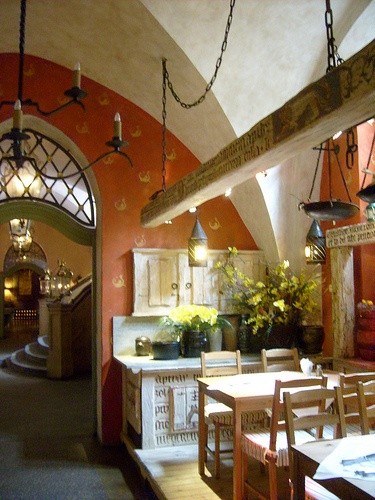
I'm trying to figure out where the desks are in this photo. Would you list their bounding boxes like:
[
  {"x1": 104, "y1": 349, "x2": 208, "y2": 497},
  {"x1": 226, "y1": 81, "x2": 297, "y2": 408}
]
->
[
  {"x1": 197, "y1": 370, "x2": 345, "y2": 500},
  {"x1": 290, "y1": 437, "x2": 375, "y2": 500},
  {"x1": 113, "y1": 354, "x2": 333, "y2": 450}
]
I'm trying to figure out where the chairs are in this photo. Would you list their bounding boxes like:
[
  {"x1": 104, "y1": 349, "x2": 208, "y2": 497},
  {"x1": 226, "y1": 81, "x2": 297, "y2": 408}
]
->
[{"x1": 194, "y1": 347, "x2": 375, "y2": 500}]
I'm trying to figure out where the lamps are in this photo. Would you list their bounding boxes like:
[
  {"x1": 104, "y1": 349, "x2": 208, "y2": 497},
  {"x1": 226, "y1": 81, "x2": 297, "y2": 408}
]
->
[
  {"x1": 186, "y1": 214, "x2": 210, "y2": 268},
  {"x1": 9, "y1": 218, "x2": 33, "y2": 259},
  {"x1": 0, "y1": 0, "x2": 135, "y2": 180},
  {"x1": 38, "y1": 257, "x2": 75, "y2": 297},
  {"x1": 304, "y1": 219, "x2": 328, "y2": 266}
]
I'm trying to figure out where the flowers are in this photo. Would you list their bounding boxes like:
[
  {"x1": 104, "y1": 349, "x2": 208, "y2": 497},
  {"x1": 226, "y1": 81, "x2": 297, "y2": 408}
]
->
[
  {"x1": 158, "y1": 303, "x2": 232, "y2": 331},
  {"x1": 211, "y1": 245, "x2": 332, "y2": 336}
]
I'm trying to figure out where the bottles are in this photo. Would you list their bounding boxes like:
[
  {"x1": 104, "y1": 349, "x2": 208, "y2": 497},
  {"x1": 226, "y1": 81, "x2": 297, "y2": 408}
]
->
[{"x1": 136, "y1": 336, "x2": 152, "y2": 356}]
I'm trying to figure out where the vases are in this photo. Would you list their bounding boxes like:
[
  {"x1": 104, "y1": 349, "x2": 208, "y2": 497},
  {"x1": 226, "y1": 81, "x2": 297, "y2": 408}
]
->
[
  {"x1": 265, "y1": 322, "x2": 293, "y2": 349},
  {"x1": 182, "y1": 328, "x2": 210, "y2": 359}
]
